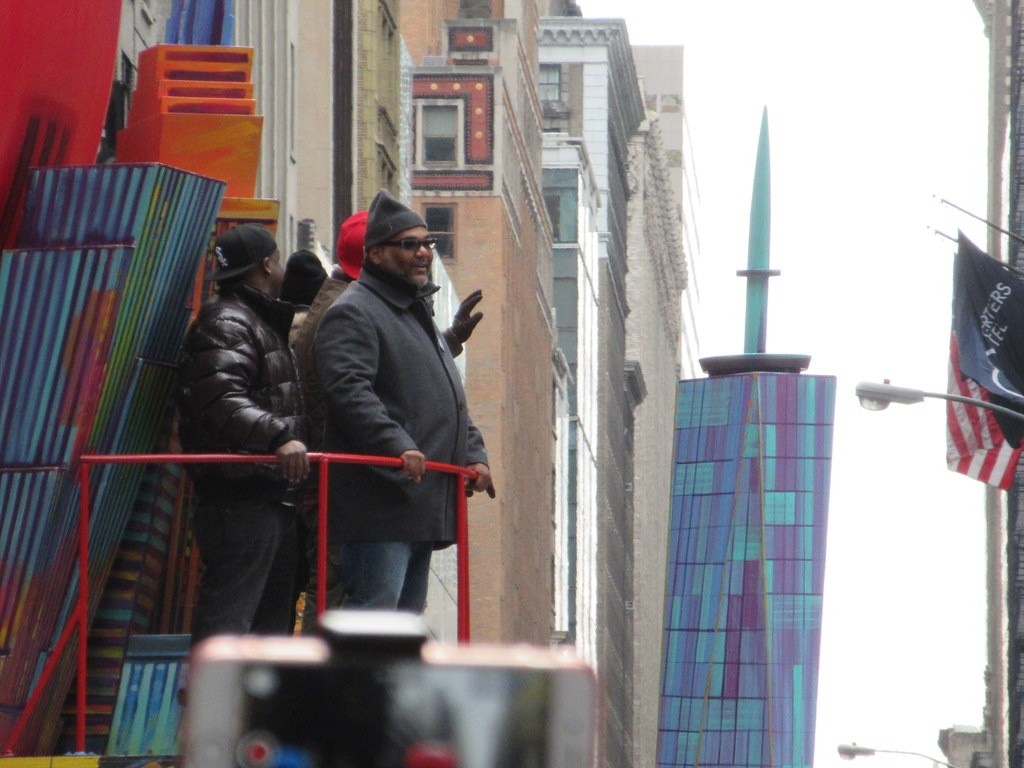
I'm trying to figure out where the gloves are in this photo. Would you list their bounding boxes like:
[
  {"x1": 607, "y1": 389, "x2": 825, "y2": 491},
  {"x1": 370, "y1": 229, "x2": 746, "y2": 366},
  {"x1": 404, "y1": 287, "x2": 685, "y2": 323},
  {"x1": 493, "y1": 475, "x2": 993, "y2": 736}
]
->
[{"x1": 451, "y1": 289, "x2": 483, "y2": 344}]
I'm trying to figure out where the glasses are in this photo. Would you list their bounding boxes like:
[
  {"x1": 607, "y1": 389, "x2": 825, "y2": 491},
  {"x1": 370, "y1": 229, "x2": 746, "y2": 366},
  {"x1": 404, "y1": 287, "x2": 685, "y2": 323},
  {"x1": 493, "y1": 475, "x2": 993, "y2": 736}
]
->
[{"x1": 387, "y1": 237, "x2": 439, "y2": 251}]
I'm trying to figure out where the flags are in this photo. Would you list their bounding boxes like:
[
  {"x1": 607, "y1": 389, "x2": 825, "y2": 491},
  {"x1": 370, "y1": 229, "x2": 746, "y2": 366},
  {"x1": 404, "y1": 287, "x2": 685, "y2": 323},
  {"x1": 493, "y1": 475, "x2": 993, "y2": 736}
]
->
[{"x1": 946, "y1": 231, "x2": 1024, "y2": 493}]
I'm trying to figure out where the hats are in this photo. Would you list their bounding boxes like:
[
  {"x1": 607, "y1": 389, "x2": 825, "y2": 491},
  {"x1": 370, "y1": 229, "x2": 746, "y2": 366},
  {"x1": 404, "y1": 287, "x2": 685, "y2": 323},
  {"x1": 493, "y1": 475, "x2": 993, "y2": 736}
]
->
[
  {"x1": 338, "y1": 210, "x2": 370, "y2": 279},
  {"x1": 204, "y1": 224, "x2": 277, "y2": 281},
  {"x1": 365, "y1": 188, "x2": 427, "y2": 251},
  {"x1": 279, "y1": 250, "x2": 329, "y2": 304}
]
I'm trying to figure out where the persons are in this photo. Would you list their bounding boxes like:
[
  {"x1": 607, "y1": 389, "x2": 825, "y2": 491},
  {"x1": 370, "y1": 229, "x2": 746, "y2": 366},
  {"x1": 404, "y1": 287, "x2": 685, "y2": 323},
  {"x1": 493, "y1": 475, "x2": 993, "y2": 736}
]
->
[{"x1": 174, "y1": 188, "x2": 492, "y2": 657}]
